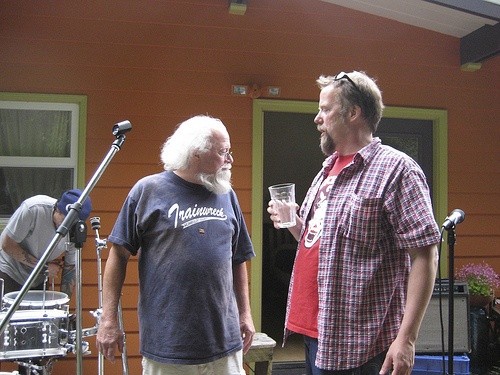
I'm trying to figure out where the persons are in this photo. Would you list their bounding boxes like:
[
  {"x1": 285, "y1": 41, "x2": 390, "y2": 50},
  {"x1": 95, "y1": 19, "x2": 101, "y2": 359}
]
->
[
  {"x1": 0, "y1": 189, "x2": 92, "y2": 295},
  {"x1": 95, "y1": 116, "x2": 257, "y2": 375},
  {"x1": 267, "y1": 71, "x2": 444, "y2": 375}
]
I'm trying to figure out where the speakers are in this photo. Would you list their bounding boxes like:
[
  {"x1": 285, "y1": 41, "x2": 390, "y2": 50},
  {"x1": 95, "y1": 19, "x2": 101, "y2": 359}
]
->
[{"x1": 414, "y1": 282, "x2": 472, "y2": 356}]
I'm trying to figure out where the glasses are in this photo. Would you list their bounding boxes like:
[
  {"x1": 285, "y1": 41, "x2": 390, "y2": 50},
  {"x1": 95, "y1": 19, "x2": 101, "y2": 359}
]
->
[
  {"x1": 216, "y1": 147, "x2": 233, "y2": 158},
  {"x1": 334, "y1": 71, "x2": 356, "y2": 88}
]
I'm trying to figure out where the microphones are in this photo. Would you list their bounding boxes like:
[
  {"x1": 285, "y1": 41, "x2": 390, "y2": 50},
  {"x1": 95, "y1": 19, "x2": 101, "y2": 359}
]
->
[{"x1": 443, "y1": 209, "x2": 466, "y2": 229}]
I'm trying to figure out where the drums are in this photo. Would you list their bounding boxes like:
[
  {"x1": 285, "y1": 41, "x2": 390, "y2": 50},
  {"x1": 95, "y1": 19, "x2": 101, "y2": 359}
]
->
[
  {"x1": 0, "y1": 308, "x2": 77, "y2": 358},
  {"x1": 2, "y1": 288, "x2": 70, "y2": 310}
]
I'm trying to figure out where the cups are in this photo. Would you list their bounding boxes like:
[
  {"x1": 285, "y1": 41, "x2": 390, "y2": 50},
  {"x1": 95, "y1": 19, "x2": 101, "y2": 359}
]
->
[{"x1": 268, "y1": 183, "x2": 297, "y2": 228}]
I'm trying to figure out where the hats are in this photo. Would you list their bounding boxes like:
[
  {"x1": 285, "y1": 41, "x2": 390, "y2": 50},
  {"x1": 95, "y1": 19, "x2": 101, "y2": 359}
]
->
[{"x1": 56, "y1": 189, "x2": 92, "y2": 220}]
